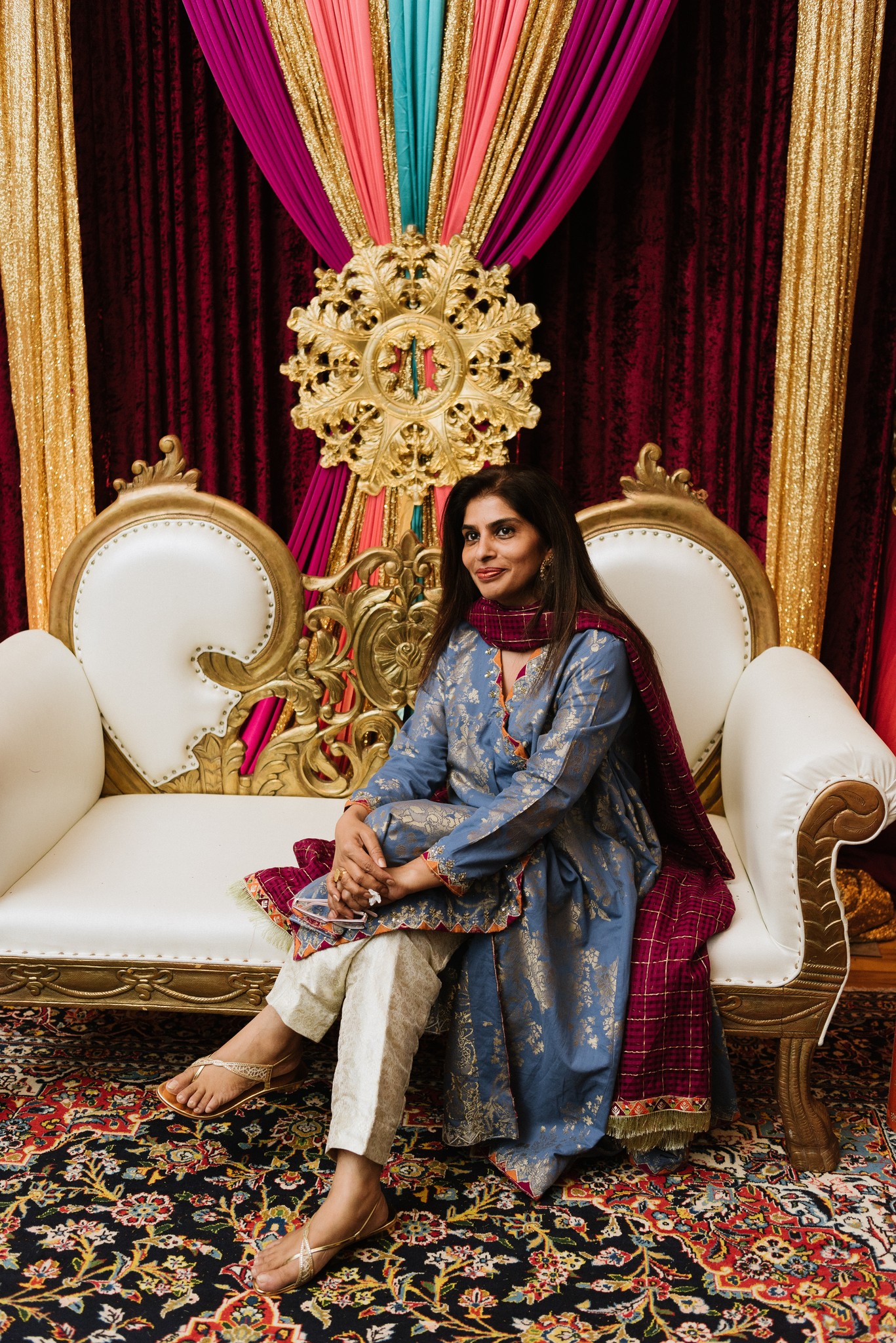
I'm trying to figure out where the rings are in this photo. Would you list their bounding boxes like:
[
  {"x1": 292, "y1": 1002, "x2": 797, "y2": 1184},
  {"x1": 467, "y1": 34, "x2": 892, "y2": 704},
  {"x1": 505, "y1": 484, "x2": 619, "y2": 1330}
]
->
[{"x1": 334, "y1": 867, "x2": 349, "y2": 882}]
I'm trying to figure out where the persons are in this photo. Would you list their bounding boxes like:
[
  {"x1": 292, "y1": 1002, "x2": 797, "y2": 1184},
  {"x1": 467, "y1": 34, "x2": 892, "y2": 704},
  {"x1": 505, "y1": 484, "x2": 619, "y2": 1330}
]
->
[{"x1": 158, "y1": 467, "x2": 737, "y2": 1293}]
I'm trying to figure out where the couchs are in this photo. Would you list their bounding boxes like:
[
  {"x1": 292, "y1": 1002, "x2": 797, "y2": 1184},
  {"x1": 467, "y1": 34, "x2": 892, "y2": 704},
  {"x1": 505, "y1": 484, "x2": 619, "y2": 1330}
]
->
[{"x1": 0, "y1": 431, "x2": 896, "y2": 1178}]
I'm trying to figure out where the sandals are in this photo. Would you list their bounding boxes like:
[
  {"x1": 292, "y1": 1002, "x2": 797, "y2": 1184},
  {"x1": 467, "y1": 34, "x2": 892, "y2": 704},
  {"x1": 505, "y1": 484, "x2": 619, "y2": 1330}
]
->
[
  {"x1": 255, "y1": 1192, "x2": 397, "y2": 1297},
  {"x1": 157, "y1": 1046, "x2": 303, "y2": 1119}
]
